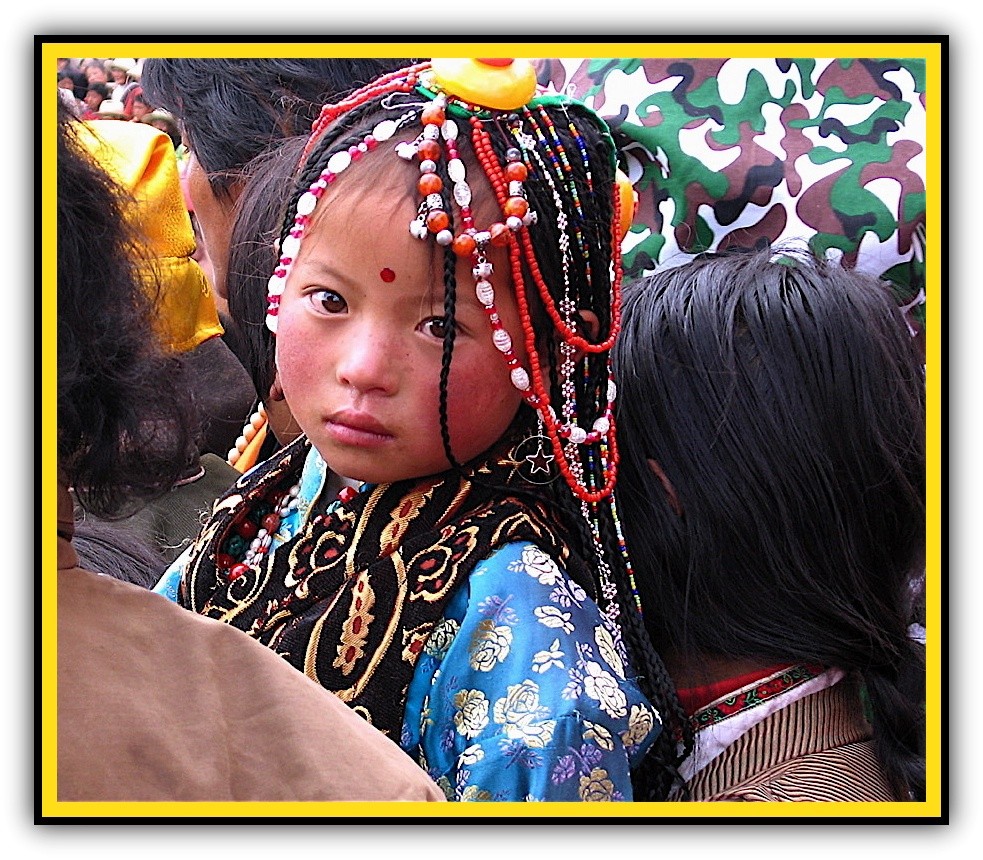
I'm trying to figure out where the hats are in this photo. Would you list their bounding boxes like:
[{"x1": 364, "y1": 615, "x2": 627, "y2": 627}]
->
[
  {"x1": 125, "y1": 58, "x2": 148, "y2": 80},
  {"x1": 103, "y1": 58, "x2": 137, "y2": 72},
  {"x1": 141, "y1": 108, "x2": 177, "y2": 133},
  {"x1": 94, "y1": 100, "x2": 131, "y2": 120}
]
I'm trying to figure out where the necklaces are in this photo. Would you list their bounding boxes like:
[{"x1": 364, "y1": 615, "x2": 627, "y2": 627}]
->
[
  {"x1": 225, "y1": 403, "x2": 270, "y2": 467},
  {"x1": 214, "y1": 469, "x2": 380, "y2": 579}
]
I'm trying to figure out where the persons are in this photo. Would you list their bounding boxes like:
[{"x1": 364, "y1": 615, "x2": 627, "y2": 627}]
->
[
  {"x1": 56, "y1": 56, "x2": 692, "y2": 803},
  {"x1": 606, "y1": 246, "x2": 926, "y2": 802}
]
